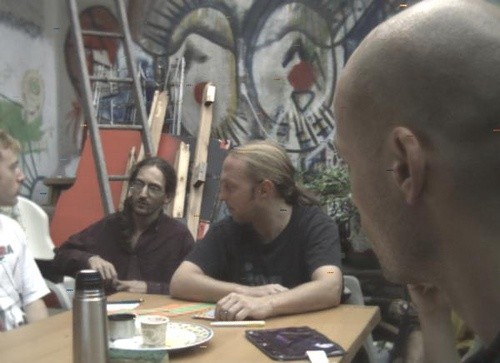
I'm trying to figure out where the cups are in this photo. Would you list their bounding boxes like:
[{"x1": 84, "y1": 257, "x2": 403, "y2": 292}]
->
[
  {"x1": 140, "y1": 314, "x2": 168, "y2": 348},
  {"x1": 107, "y1": 314, "x2": 136, "y2": 340}
]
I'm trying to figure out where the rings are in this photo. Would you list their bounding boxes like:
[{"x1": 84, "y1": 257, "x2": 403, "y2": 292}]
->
[{"x1": 220, "y1": 311, "x2": 227, "y2": 314}]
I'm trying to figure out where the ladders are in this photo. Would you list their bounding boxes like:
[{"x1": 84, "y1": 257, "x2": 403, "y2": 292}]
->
[{"x1": 68, "y1": 0, "x2": 157, "y2": 216}]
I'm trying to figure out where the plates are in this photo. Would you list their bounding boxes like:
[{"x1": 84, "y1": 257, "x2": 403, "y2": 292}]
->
[{"x1": 108, "y1": 322, "x2": 214, "y2": 352}]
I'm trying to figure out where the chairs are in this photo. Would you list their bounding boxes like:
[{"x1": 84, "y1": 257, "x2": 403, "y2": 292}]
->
[
  {"x1": 340, "y1": 273, "x2": 390, "y2": 363},
  {"x1": 9, "y1": 192, "x2": 77, "y2": 310}
]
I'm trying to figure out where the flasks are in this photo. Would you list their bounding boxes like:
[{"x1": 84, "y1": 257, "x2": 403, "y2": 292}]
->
[{"x1": 71, "y1": 269, "x2": 108, "y2": 363}]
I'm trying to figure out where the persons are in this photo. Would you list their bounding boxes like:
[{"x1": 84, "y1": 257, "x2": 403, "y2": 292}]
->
[
  {"x1": 56, "y1": 155, "x2": 195, "y2": 296},
  {"x1": 331, "y1": 0, "x2": 500, "y2": 363},
  {"x1": 0, "y1": 134, "x2": 52, "y2": 332},
  {"x1": 169, "y1": 140, "x2": 343, "y2": 321}
]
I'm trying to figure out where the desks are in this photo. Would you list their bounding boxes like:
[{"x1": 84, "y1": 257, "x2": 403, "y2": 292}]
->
[{"x1": 0, "y1": 283, "x2": 381, "y2": 363}]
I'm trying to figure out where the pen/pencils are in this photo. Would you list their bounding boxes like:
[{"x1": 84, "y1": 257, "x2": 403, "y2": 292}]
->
[
  {"x1": 210, "y1": 320, "x2": 265, "y2": 328},
  {"x1": 107, "y1": 298, "x2": 144, "y2": 304}
]
